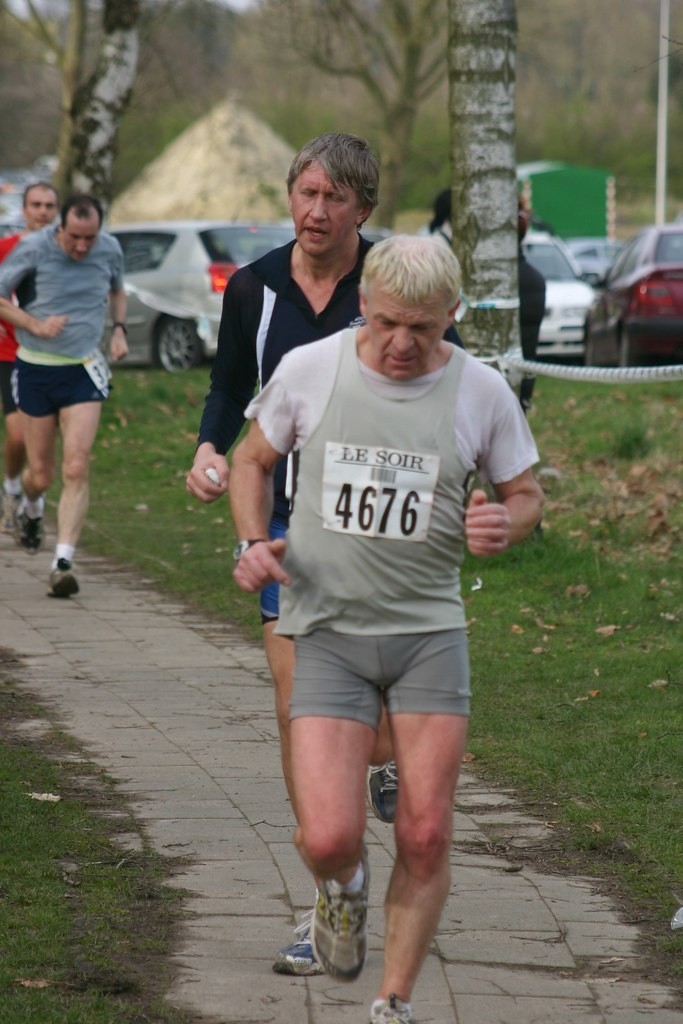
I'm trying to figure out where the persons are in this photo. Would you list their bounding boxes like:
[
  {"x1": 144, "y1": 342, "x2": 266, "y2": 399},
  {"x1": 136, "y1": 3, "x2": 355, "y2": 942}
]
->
[
  {"x1": 0, "y1": 181, "x2": 128, "y2": 598},
  {"x1": 419, "y1": 187, "x2": 547, "y2": 416},
  {"x1": 183, "y1": 136, "x2": 398, "y2": 977},
  {"x1": 224, "y1": 232, "x2": 548, "y2": 1024}
]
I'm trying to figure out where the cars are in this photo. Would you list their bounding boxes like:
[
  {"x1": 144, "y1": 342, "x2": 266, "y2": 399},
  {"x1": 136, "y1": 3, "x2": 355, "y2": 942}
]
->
[
  {"x1": 98, "y1": 222, "x2": 298, "y2": 373},
  {"x1": 521, "y1": 228, "x2": 628, "y2": 356},
  {"x1": 576, "y1": 225, "x2": 683, "y2": 368}
]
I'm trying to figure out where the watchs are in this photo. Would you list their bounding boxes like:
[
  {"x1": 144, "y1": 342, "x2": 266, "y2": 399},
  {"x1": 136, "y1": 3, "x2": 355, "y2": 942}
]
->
[{"x1": 233, "y1": 539, "x2": 266, "y2": 561}]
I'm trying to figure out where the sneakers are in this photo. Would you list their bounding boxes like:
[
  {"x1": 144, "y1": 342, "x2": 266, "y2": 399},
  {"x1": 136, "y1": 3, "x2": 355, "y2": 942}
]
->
[
  {"x1": 312, "y1": 845, "x2": 367, "y2": 983},
  {"x1": 272, "y1": 887, "x2": 326, "y2": 975},
  {"x1": 366, "y1": 758, "x2": 399, "y2": 822},
  {"x1": 20, "y1": 495, "x2": 45, "y2": 555},
  {"x1": 47, "y1": 558, "x2": 80, "y2": 598},
  {"x1": 0, "y1": 480, "x2": 22, "y2": 533},
  {"x1": 370, "y1": 993, "x2": 411, "y2": 1024}
]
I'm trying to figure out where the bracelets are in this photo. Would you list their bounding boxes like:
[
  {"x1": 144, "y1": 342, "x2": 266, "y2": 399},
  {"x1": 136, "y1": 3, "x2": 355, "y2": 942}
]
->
[{"x1": 114, "y1": 322, "x2": 127, "y2": 335}]
